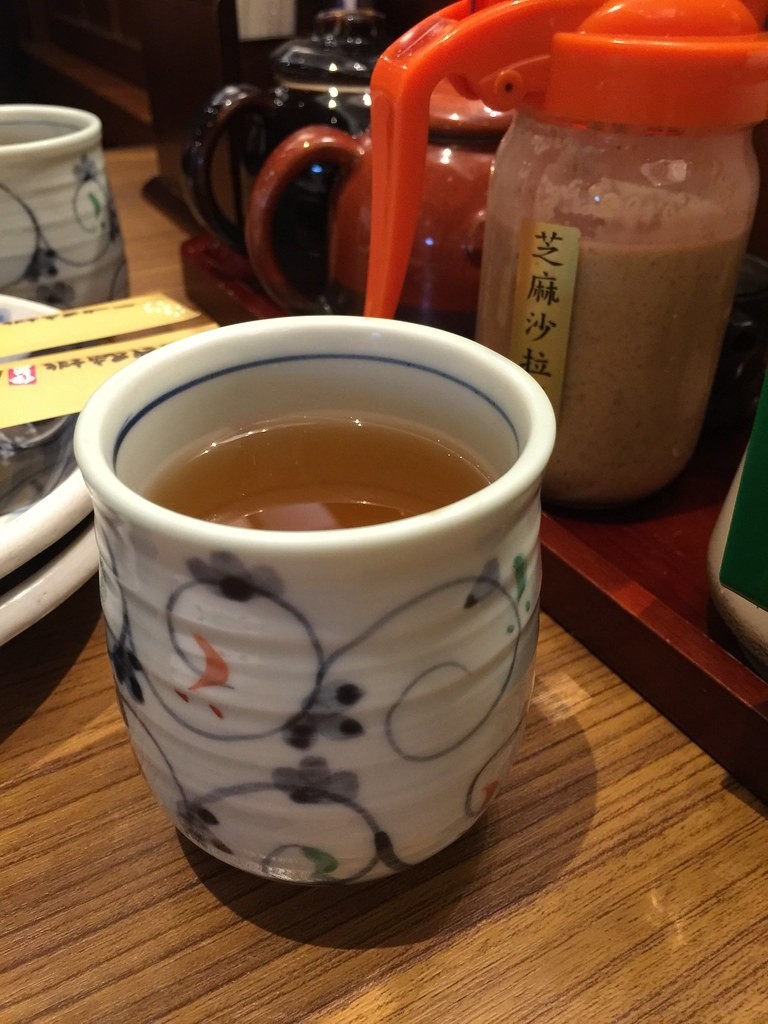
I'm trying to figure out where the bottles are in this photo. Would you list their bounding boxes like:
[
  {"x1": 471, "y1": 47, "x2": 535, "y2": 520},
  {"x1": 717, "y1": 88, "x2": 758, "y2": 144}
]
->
[{"x1": 357, "y1": 3, "x2": 767, "y2": 512}]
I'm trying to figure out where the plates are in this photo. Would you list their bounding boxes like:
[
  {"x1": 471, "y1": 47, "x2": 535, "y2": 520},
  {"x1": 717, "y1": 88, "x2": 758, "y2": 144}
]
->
[{"x1": 2, "y1": 289, "x2": 131, "y2": 645}]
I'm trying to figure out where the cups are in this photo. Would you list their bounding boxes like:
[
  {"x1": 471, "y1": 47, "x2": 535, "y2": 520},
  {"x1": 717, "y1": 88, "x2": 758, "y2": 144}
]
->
[
  {"x1": 4, "y1": 101, "x2": 126, "y2": 313},
  {"x1": 74, "y1": 304, "x2": 570, "y2": 890}
]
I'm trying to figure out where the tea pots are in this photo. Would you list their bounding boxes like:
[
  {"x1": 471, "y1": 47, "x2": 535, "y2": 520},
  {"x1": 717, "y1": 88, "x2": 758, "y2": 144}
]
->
[{"x1": 170, "y1": 7, "x2": 515, "y2": 330}]
